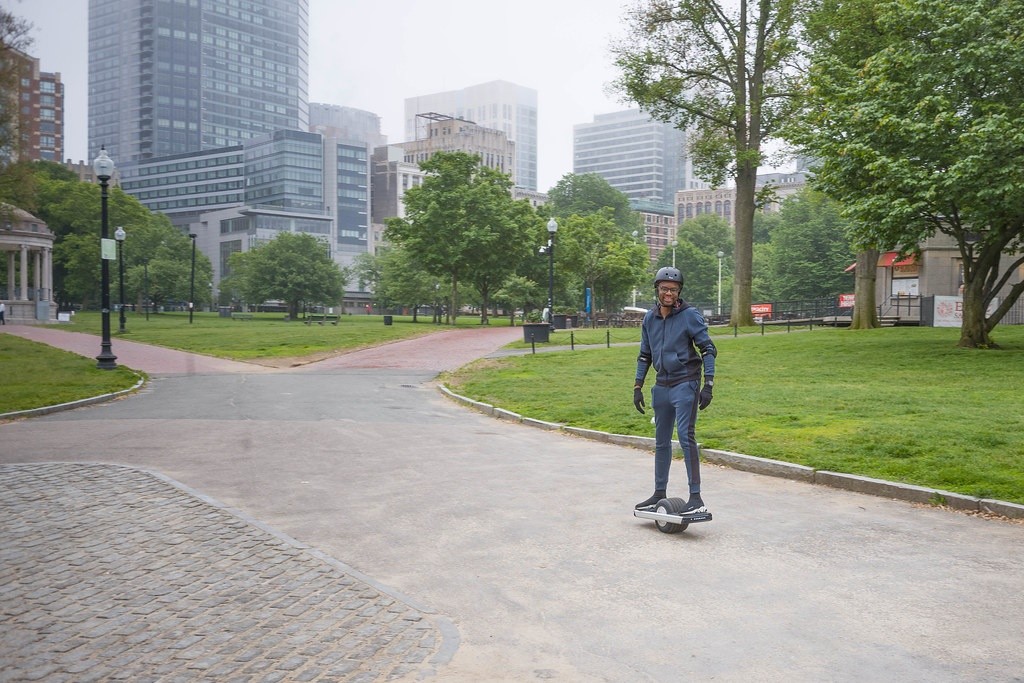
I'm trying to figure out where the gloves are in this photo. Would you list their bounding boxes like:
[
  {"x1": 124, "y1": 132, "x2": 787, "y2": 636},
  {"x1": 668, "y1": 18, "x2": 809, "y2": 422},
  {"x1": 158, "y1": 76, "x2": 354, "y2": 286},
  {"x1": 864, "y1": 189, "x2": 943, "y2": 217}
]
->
[
  {"x1": 699, "y1": 385, "x2": 714, "y2": 410},
  {"x1": 633, "y1": 388, "x2": 646, "y2": 414}
]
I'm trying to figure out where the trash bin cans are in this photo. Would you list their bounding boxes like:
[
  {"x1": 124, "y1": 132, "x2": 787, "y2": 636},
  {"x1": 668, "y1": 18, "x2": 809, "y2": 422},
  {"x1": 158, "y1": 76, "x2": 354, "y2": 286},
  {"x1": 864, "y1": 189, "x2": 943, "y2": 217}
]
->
[
  {"x1": 219, "y1": 306, "x2": 231, "y2": 317},
  {"x1": 384, "y1": 316, "x2": 391, "y2": 325}
]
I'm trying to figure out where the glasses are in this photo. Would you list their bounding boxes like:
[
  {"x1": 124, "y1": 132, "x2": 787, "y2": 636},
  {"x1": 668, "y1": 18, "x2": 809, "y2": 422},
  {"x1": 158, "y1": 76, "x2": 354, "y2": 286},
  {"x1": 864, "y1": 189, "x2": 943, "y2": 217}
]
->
[{"x1": 661, "y1": 286, "x2": 682, "y2": 293}]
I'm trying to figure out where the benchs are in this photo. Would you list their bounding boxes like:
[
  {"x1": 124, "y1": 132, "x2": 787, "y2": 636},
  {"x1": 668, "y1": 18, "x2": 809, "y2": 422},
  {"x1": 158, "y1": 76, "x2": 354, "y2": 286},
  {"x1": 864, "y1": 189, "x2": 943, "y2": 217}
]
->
[
  {"x1": 302, "y1": 314, "x2": 341, "y2": 325},
  {"x1": 231, "y1": 313, "x2": 256, "y2": 321}
]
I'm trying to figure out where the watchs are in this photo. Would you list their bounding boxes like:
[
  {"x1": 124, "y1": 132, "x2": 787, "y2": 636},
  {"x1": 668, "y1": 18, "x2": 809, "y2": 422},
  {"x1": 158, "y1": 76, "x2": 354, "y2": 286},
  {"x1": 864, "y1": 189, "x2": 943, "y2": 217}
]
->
[{"x1": 705, "y1": 380, "x2": 714, "y2": 386}]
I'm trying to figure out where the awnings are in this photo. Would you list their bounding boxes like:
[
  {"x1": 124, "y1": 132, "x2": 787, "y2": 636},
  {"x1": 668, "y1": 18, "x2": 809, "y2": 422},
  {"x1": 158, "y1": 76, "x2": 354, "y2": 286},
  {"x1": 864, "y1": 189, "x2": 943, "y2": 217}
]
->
[
  {"x1": 845, "y1": 262, "x2": 857, "y2": 272},
  {"x1": 876, "y1": 253, "x2": 919, "y2": 268}
]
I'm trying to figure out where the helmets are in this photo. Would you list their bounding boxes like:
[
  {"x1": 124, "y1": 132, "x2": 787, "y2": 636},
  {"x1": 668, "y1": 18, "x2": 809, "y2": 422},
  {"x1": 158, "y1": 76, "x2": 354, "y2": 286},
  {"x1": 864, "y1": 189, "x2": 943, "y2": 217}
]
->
[{"x1": 654, "y1": 267, "x2": 684, "y2": 289}]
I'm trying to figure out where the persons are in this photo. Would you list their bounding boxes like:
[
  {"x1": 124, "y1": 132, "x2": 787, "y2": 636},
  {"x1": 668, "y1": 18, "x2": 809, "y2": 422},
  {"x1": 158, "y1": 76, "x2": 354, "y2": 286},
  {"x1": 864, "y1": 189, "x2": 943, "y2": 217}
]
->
[
  {"x1": 542, "y1": 305, "x2": 553, "y2": 323},
  {"x1": 634, "y1": 267, "x2": 718, "y2": 515},
  {"x1": 0, "y1": 301, "x2": 6, "y2": 325}
]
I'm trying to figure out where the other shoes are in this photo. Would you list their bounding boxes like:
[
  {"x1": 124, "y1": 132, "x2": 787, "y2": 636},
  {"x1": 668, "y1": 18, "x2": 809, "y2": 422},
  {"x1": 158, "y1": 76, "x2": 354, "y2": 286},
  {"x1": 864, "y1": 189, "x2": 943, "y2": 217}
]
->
[
  {"x1": 635, "y1": 496, "x2": 667, "y2": 510},
  {"x1": 678, "y1": 499, "x2": 707, "y2": 515}
]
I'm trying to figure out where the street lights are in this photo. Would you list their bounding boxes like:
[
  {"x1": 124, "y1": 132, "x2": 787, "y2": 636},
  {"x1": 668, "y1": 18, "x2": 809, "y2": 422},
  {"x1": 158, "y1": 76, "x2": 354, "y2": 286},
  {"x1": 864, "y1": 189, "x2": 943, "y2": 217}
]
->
[
  {"x1": 716, "y1": 250, "x2": 725, "y2": 316},
  {"x1": 435, "y1": 282, "x2": 441, "y2": 324},
  {"x1": 671, "y1": 240, "x2": 679, "y2": 268},
  {"x1": 91, "y1": 143, "x2": 119, "y2": 371},
  {"x1": 631, "y1": 230, "x2": 639, "y2": 308},
  {"x1": 188, "y1": 233, "x2": 197, "y2": 324},
  {"x1": 114, "y1": 226, "x2": 130, "y2": 334},
  {"x1": 537, "y1": 216, "x2": 558, "y2": 332}
]
